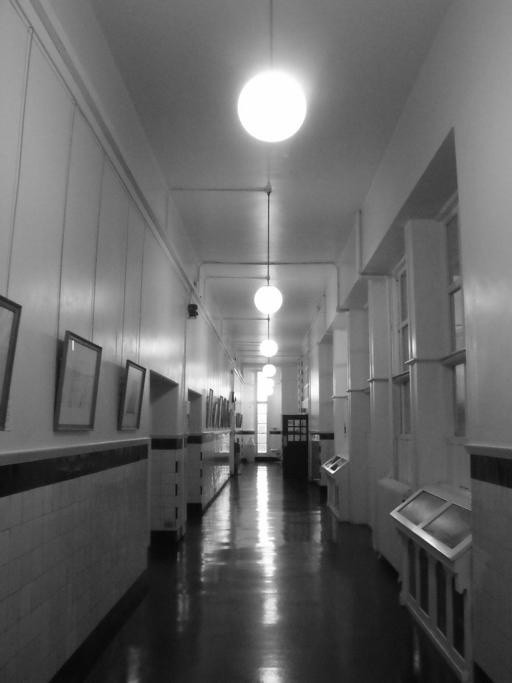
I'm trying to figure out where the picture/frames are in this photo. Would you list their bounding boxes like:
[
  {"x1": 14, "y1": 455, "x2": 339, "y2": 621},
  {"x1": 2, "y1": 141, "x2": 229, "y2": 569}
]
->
[
  {"x1": 206, "y1": 388, "x2": 243, "y2": 429},
  {"x1": 0, "y1": 295, "x2": 146, "y2": 432}
]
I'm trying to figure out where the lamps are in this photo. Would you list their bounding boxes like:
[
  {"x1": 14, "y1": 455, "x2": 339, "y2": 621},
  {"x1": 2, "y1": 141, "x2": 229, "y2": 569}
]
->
[{"x1": 236, "y1": 0, "x2": 308, "y2": 380}]
